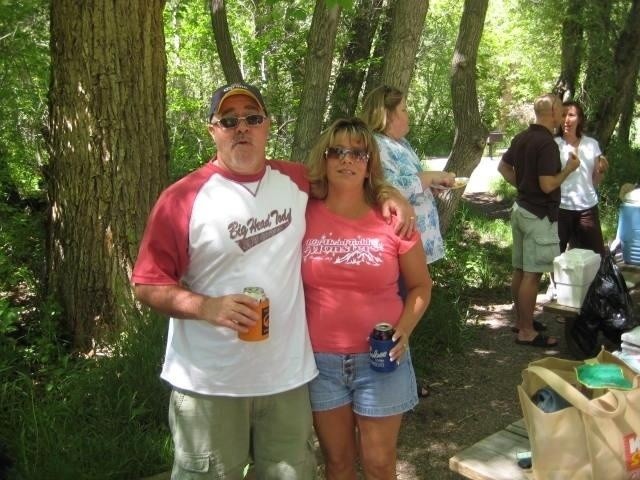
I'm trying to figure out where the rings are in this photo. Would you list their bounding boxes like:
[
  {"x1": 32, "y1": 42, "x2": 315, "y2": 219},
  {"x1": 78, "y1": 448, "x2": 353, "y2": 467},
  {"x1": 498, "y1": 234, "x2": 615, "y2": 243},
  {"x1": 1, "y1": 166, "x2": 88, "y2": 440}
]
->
[
  {"x1": 404, "y1": 344, "x2": 409, "y2": 354},
  {"x1": 410, "y1": 217, "x2": 417, "y2": 224}
]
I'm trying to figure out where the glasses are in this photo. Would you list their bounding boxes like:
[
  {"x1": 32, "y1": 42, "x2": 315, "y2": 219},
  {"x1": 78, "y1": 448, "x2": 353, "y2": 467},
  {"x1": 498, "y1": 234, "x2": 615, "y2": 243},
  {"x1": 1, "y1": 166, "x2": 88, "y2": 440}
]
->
[
  {"x1": 215, "y1": 115, "x2": 266, "y2": 130},
  {"x1": 384, "y1": 84, "x2": 391, "y2": 107},
  {"x1": 324, "y1": 147, "x2": 370, "y2": 162}
]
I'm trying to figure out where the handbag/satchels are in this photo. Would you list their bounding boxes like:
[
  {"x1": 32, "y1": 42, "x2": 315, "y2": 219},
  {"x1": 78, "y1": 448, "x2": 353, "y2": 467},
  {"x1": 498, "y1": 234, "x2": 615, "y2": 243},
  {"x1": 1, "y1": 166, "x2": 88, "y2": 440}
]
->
[{"x1": 518, "y1": 345, "x2": 640, "y2": 480}]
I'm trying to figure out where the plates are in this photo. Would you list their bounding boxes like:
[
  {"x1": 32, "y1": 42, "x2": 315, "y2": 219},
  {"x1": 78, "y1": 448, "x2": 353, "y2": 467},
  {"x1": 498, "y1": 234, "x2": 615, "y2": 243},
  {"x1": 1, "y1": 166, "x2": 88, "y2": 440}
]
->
[{"x1": 430, "y1": 177, "x2": 470, "y2": 190}]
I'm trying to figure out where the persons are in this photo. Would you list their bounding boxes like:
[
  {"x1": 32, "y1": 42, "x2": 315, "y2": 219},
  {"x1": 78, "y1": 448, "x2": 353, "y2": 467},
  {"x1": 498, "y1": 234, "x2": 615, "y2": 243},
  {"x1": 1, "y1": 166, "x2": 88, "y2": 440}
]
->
[
  {"x1": 301, "y1": 117, "x2": 433, "y2": 480},
  {"x1": 553, "y1": 100, "x2": 609, "y2": 261},
  {"x1": 131, "y1": 83, "x2": 416, "y2": 479},
  {"x1": 358, "y1": 85, "x2": 456, "y2": 398},
  {"x1": 497, "y1": 93, "x2": 580, "y2": 346}
]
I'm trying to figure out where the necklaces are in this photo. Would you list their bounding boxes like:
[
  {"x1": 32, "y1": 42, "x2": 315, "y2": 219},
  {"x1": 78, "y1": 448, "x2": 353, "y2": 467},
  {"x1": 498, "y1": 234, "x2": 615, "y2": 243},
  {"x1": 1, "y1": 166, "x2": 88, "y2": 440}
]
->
[{"x1": 226, "y1": 172, "x2": 265, "y2": 198}]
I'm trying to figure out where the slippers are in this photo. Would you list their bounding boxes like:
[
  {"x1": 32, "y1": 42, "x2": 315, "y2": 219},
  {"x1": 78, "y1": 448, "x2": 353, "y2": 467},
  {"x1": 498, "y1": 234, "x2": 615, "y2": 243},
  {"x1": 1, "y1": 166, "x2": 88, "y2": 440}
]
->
[
  {"x1": 515, "y1": 333, "x2": 558, "y2": 346},
  {"x1": 511, "y1": 320, "x2": 547, "y2": 332}
]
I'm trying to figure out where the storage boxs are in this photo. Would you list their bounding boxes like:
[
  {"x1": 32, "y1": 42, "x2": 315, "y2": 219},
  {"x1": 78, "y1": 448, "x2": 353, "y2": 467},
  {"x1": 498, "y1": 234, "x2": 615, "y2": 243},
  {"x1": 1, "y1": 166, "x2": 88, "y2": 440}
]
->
[{"x1": 543, "y1": 270, "x2": 639, "y2": 347}]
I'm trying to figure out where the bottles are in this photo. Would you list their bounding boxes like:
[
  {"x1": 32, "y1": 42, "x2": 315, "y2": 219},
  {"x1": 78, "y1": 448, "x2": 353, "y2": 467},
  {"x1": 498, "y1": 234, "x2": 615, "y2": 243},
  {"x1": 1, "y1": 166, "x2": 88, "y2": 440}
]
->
[
  {"x1": 239, "y1": 287, "x2": 269, "y2": 341},
  {"x1": 369, "y1": 323, "x2": 398, "y2": 372}
]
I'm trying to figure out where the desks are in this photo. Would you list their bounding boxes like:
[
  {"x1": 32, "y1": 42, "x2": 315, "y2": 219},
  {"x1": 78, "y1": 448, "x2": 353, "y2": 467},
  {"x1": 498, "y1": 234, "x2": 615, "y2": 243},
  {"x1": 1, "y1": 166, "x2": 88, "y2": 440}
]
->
[{"x1": 448, "y1": 415, "x2": 534, "y2": 480}]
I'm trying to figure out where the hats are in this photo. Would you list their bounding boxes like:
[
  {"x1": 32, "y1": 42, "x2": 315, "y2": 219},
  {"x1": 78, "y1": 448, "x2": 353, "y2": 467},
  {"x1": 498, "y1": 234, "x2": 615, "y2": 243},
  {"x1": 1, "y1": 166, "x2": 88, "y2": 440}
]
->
[{"x1": 211, "y1": 83, "x2": 266, "y2": 117}]
